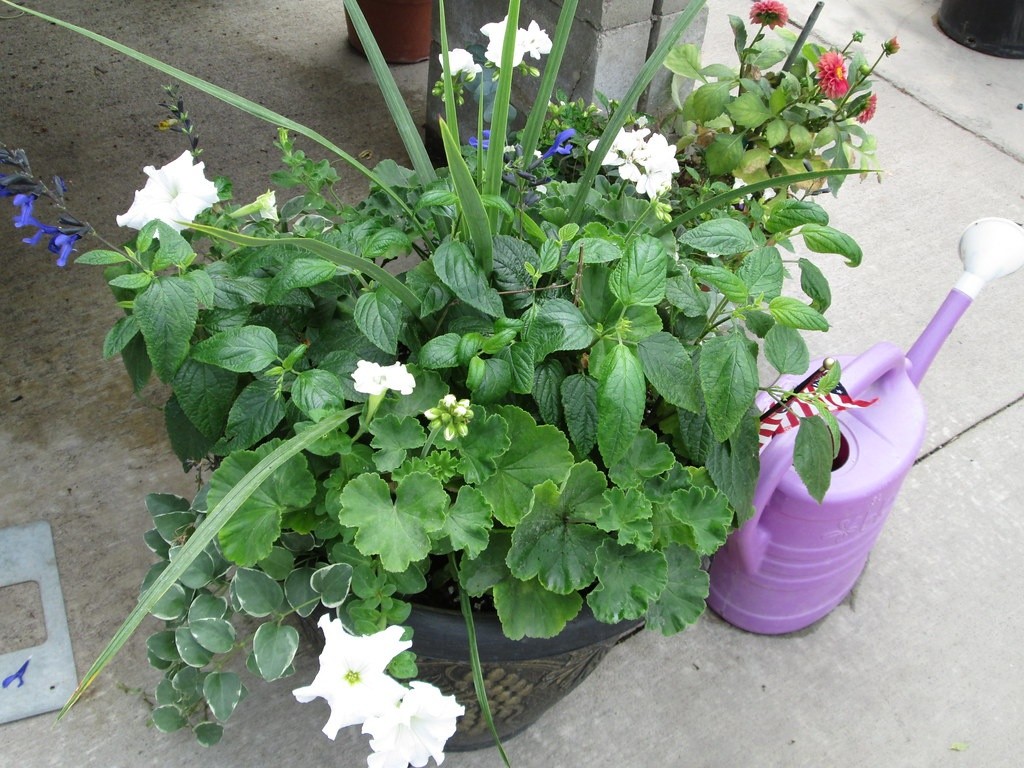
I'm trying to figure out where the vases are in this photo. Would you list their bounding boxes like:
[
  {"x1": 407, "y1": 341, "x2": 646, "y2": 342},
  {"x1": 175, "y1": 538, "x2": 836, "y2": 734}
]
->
[{"x1": 294, "y1": 555, "x2": 711, "y2": 755}]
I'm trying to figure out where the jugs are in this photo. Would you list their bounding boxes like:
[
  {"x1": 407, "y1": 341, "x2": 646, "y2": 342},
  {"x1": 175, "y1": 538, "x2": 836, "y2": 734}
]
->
[{"x1": 707, "y1": 218, "x2": 1024, "y2": 635}]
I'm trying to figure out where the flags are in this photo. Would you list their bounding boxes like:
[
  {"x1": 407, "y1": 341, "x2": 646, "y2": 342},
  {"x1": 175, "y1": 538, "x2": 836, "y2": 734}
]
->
[{"x1": 759, "y1": 365, "x2": 879, "y2": 456}]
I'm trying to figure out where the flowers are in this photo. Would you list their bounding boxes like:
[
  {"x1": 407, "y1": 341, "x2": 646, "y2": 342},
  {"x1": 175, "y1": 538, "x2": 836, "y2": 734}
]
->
[{"x1": 0, "y1": 0, "x2": 899, "y2": 768}]
